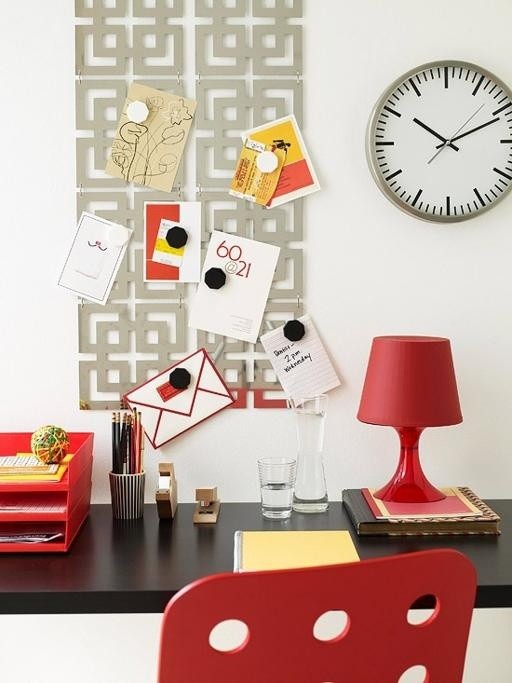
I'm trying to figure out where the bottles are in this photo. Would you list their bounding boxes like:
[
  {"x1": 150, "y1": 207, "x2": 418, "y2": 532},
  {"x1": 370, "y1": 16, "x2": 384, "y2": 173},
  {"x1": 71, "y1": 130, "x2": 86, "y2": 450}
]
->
[{"x1": 292, "y1": 392, "x2": 328, "y2": 515}]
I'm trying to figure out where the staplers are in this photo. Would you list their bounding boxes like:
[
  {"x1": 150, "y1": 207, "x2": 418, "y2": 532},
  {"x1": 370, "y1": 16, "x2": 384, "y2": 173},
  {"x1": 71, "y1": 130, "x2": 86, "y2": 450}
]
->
[{"x1": 193, "y1": 486, "x2": 221, "y2": 524}]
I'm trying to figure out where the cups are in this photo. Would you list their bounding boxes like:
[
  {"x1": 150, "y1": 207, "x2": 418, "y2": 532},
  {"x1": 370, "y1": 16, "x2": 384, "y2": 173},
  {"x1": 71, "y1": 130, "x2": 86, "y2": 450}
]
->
[
  {"x1": 108, "y1": 470, "x2": 146, "y2": 523},
  {"x1": 256, "y1": 457, "x2": 298, "y2": 520}
]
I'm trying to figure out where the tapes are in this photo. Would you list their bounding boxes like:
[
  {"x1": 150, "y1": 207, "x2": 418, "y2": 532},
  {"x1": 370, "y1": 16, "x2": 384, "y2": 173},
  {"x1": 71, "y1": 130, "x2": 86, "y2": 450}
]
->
[{"x1": 159, "y1": 475, "x2": 171, "y2": 490}]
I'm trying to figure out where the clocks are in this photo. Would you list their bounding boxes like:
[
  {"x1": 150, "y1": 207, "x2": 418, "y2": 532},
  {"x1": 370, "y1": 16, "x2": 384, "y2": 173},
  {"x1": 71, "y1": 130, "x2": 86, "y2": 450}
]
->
[{"x1": 364, "y1": 60, "x2": 511, "y2": 225}]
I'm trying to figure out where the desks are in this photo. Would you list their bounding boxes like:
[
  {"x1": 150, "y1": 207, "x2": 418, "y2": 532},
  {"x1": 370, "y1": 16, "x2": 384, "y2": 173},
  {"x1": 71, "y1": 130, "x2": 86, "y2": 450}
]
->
[{"x1": 1, "y1": 498, "x2": 512, "y2": 615}]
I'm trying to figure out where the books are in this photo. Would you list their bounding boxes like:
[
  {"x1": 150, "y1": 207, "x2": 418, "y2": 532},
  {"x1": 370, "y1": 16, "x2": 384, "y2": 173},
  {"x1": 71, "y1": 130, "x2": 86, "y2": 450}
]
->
[
  {"x1": 341, "y1": 484, "x2": 504, "y2": 538},
  {"x1": 233, "y1": 529, "x2": 362, "y2": 573},
  {"x1": 0, "y1": 452, "x2": 75, "y2": 485}
]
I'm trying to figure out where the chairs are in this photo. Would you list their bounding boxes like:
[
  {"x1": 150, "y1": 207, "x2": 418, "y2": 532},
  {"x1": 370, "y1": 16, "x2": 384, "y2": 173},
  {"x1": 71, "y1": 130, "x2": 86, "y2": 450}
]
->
[{"x1": 157, "y1": 548, "x2": 478, "y2": 682}]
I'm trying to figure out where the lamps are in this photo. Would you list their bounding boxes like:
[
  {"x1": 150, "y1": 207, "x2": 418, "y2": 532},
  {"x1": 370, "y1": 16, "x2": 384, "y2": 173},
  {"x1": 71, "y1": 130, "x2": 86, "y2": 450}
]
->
[{"x1": 358, "y1": 336, "x2": 464, "y2": 503}]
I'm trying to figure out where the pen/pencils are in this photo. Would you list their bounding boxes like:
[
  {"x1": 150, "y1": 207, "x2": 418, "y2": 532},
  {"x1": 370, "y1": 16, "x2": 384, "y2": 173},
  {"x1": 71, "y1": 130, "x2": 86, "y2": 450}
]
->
[{"x1": 111, "y1": 407, "x2": 142, "y2": 474}]
